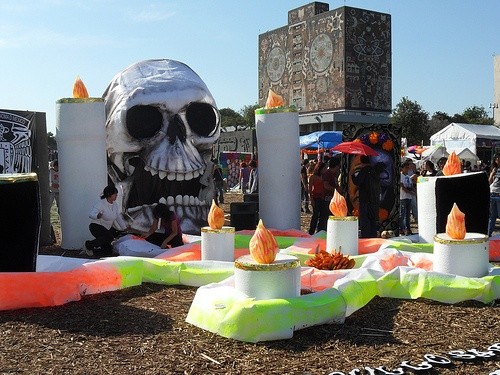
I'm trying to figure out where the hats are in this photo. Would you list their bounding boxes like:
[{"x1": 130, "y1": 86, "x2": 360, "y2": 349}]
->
[
  {"x1": 100, "y1": 185, "x2": 118, "y2": 200},
  {"x1": 154, "y1": 203, "x2": 169, "y2": 217}
]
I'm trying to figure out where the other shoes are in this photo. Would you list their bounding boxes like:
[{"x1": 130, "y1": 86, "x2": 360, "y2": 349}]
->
[{"x1": 83, "y1": 239, "x2": 94, "y2": 256}]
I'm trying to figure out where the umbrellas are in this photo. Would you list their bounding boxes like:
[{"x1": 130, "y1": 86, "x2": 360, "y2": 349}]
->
[{"x1": 333, "y1": 138, "x2": 379, "y2": 165}]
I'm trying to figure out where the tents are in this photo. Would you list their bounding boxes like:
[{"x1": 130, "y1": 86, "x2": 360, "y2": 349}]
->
[
  {"x1": 299, "y1": 131, "x2": 343, "y2": 149},
  {"x1": 430, "y1": 123, "x2": 500, "y2": 159}
]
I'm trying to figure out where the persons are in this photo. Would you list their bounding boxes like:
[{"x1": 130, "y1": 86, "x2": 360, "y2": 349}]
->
[
  {"x1": 84, "y1": 185, "x2": 128, "y2": 257},
  {"x1": 48, "y1": 159, "x2": 60, "y2": 216},
  {"x1": 300, "y1": 155, "x2": 344, "y2": 235},
  {"x1": 239, "y1": 160, "x2": 258, "y2": 194},
  {"x1": 399, "y1": 153, "x2": 500, "y2": 238},
  {"x1": 141, "y1": 203, "x2": 183, "y2": 249},
  {"x1": 351, "y1": 155, "x2": 387, "y2": 238},
  {"x1": 212, "y1": 158, "x2": 224, "y2": 205}
]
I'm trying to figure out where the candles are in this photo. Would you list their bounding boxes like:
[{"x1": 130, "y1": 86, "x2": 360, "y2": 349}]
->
[
  {"x1": 433, "y1": 202, "x2": 490, "y2": 278},
  {"x1": 201, "y1": 198, "x2": 235, "y2": 262},
  {"x1": 236, "y1": 221, "x2": 301, "y2": 302},
  {"x1": 326, "y1": 188, "x2": 358, "y2": 255},
  {"x1": 255, "y1": 88, "x2": 302, "y2": 230}
]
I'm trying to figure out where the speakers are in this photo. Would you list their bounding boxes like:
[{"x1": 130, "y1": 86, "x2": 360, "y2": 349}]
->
[{"x1": 229, "y1": 202, "x2": 259, "y2": 231}]
